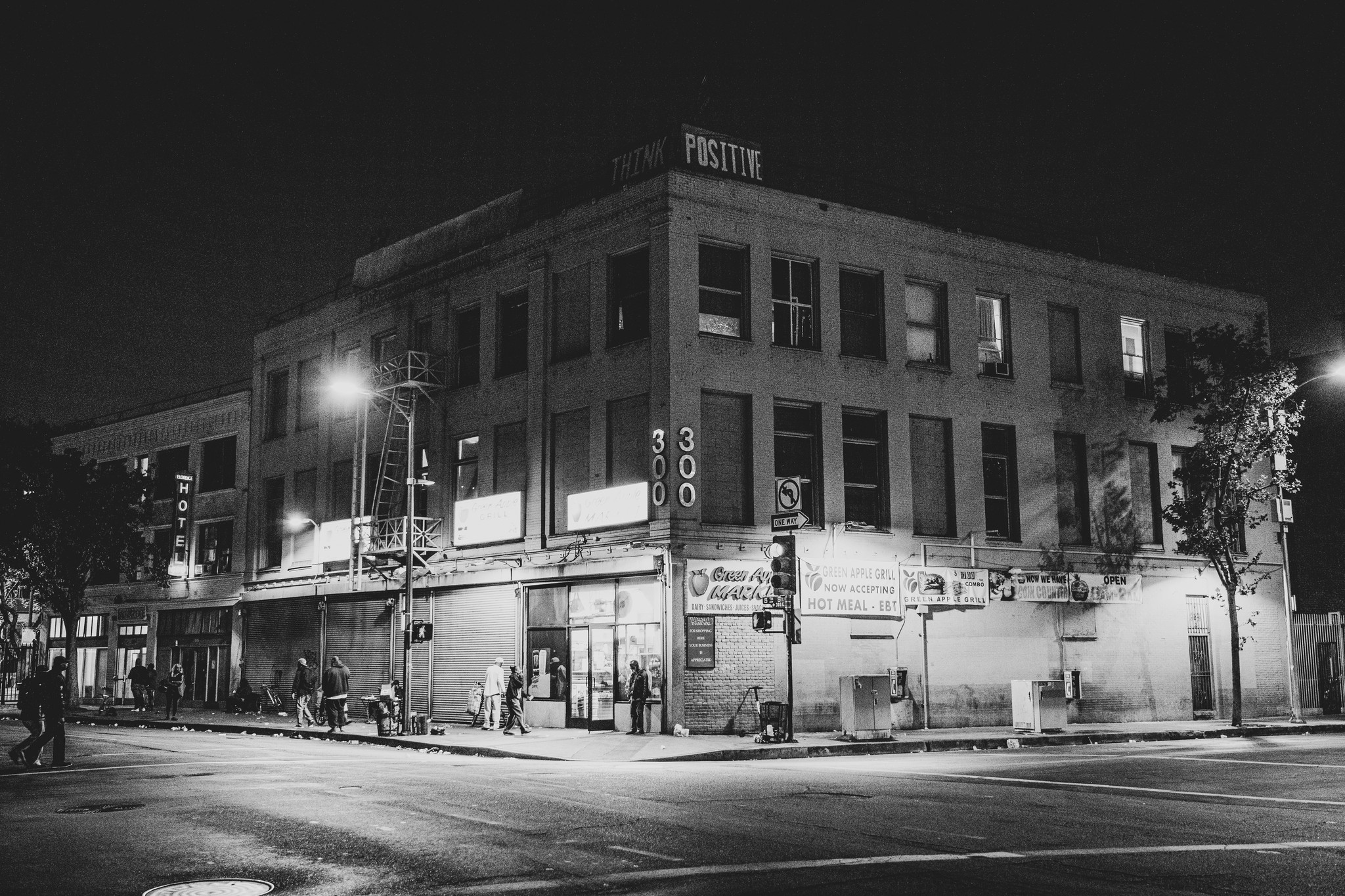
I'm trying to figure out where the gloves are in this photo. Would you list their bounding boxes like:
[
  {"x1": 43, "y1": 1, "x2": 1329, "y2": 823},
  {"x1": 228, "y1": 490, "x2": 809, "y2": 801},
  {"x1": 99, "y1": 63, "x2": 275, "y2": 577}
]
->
[{"x1": 291, "y1": 693, "x2": 295, "y2": 699}]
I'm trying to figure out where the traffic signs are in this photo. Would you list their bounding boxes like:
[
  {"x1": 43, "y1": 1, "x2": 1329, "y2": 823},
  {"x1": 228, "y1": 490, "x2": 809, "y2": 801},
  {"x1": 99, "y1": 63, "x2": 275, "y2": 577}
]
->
[{"x1": 771, "y1": 511, "x2": 809, "y2": 533}]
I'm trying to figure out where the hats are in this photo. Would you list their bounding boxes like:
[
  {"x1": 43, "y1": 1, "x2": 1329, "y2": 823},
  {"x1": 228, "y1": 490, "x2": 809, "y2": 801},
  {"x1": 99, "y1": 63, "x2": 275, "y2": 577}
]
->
[
  {"x1": 391, "y1": 680, "x2": 399, "y2": 685},
  {"x1": 298, "y1": 658, "x2": 308, "y2": 666},
  {"x1": 496, "y1": 657, "x2": 504, "y2": 664},
  {"x1": 549, "y1": 657, "x2": 560, "y2": 664},
  {"x1": 147, "y1": 663, "x2": 155, "y2": 668}
]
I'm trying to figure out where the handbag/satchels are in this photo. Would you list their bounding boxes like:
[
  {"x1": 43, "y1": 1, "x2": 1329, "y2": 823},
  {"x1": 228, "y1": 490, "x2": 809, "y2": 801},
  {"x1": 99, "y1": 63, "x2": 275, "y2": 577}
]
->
[
  {"x1": 158, "y1": 671, "x2": 170, "y2": 693},
  {"x1": 465, "y1": 680, "x2": 481, "y2": 715},
  {"x1": 431, "y1": 727, "x2": 446, "y2": 735}
]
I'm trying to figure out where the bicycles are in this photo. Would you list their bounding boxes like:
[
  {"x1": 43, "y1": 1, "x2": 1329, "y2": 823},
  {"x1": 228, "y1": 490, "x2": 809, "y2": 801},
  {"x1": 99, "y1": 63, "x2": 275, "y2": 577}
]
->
[
  {"x1": 471, "y1": 680, "x2": 510, "y2": 729},
  {"x1": 255, "y1": 680, "x2": 285, "y2": 714},
  {"x1": 98, "y1": 693, "x2": 116, "y2": 716}
]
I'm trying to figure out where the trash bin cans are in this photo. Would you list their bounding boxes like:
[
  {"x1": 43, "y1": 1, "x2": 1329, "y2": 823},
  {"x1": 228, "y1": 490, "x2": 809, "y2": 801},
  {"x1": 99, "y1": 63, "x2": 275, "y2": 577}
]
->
[
  {"x1": 411, "y1": 714, "x2": 428, "y2": 735},
  {"x1": 375, "y1": 698, "x2": 402, "y2": 736},
  {"x1": 758, "y1": 700, "x2": 790, "y2": 743}
]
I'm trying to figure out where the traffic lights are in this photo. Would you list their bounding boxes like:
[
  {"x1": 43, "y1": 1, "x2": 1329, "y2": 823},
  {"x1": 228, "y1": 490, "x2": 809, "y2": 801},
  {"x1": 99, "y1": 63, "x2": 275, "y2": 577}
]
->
[
  {"x1": 752, "y1": 610, "x2": 772, "y2": 630},
  {"x1": 769, "y1": 513, "x2": 796, "y2": 595}
]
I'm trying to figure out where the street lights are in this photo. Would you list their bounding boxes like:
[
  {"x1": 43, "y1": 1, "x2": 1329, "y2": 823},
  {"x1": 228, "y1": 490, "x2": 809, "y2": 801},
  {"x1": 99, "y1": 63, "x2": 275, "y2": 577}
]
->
[
  {"x1": 1270, "y1": 366, "x2": 1345, "y2": 724},
  {"x1": 327, "y1": 375, "x2": 416, "y2": 736}
]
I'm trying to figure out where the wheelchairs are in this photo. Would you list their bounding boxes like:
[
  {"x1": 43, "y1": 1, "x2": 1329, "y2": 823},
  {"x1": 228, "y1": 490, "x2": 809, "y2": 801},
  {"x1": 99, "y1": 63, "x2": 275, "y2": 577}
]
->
[{"x1": 314, "y1": 692, "x2": 328, "y2": 726}]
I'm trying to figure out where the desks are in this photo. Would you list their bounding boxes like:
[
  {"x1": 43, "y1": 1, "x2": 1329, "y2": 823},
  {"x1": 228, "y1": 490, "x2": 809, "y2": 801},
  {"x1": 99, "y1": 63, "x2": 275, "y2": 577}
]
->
[{"x1": 354, "y1": 697, "x2": 380, "y2": 726}]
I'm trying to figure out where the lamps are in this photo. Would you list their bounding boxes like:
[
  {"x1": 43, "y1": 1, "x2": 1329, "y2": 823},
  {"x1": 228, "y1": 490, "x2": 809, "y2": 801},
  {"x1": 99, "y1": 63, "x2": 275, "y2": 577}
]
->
[{"x1": 301, "y1": 518, "x2": 320, "y2": 530}]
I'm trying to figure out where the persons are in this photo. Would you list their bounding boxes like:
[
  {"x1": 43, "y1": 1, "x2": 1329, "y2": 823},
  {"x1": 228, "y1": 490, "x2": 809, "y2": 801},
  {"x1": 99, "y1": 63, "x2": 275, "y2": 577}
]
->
[
  {"x1": 163, "y1": 662, "x2": 183, "y2": 722},
  {"x1": 626, "y1": 660, "x2": 649, "y2": 736},
  {"x1": 127, "y1": 658, "x2": 157, "y2": 713},
  {"x1": 418, "y1": 626, "x2": 426, "y2": 639},
  {"x1": 232, "y1": 678, "x2": 252, "y2": 715},
  {"x1": 503, "y1": 664, "x2": 533, "y2": 736},
  {"x1": 391, "y1": 680, "x2": 403, "y2": 699},
  {"x1": 291, "y1": 659, "x2": 314, "y2": 727},
  {"x1": 322, "y1": 656, "x2": 353, "y2": 735},
  {"x1": 481, "y1": 657, "x2": 506, "y2": 731},
  {"x1": 549, "y1": 657, "x2": 566, "y2": 700},
  {"x1": 8, "y1": 656, "x2": 75, "y2": 770}
]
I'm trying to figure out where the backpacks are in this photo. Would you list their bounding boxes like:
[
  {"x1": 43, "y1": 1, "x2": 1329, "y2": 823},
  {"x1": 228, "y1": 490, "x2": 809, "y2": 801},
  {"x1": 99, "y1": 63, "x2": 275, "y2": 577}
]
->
[
  {"x1": 17, "y1": 675, "x2": 32, "y2": 710},
  {"x1": 297, "y1": 666, "x2": 317, "y2": 702}
]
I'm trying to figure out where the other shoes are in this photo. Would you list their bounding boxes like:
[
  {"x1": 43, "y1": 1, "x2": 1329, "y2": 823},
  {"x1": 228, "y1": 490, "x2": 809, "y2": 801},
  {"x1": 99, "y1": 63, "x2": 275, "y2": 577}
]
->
[
  {"x1": 481, "y1": 726, "x2": 490, "y2": 730},
  {"x1": 8, "y1": 750, "x2": 21, "y2": 765},
  {"x1": 165, "y1": 716, "x2": 169, "y2": 720},
  {"x1": 51, "y1": 761, "x2": 73, "y2": 768},
  {"x1": 625, "y1": 730, "x2": 637, "y2": 734},
  {"x1": 493, "y1": 726, "x2": 504, "y2": 730},
  {"x1": 340, "y1": 726, "x2": 345, "y2": 732},
  {"x1": 20, "y1": 750, "x2": 33, "y2": 768},
  {"x1": 504, "y1": 731, "x2": 515, "y2": 735},
  {"x1": 131, "y1": 707, "x2": 146, "y2": 711},
  {"x1": 328, "y1": 728, "x2": 338, "y2": 733},
  {"x1": 294, "y1": 725, "x2": 304, "y2": 728},
  {"x1": 28, "y1": 763, "x2": 46, "y2": 769},
  {"x1": 521, "y1": 729, "x2": 532, "y2": 735},
  {"x1": 366, "y1": 717, "x2": 376, "y2": 723},
  {"x1": 308, "y1": 719, "x2": 314, "y2": 726},
  {"x1": 633, "y1": 730, "x2": 645, "y2": 735},
  {"x1": 171, "y1": 717, "x2": 178, "y2": 720}
]
